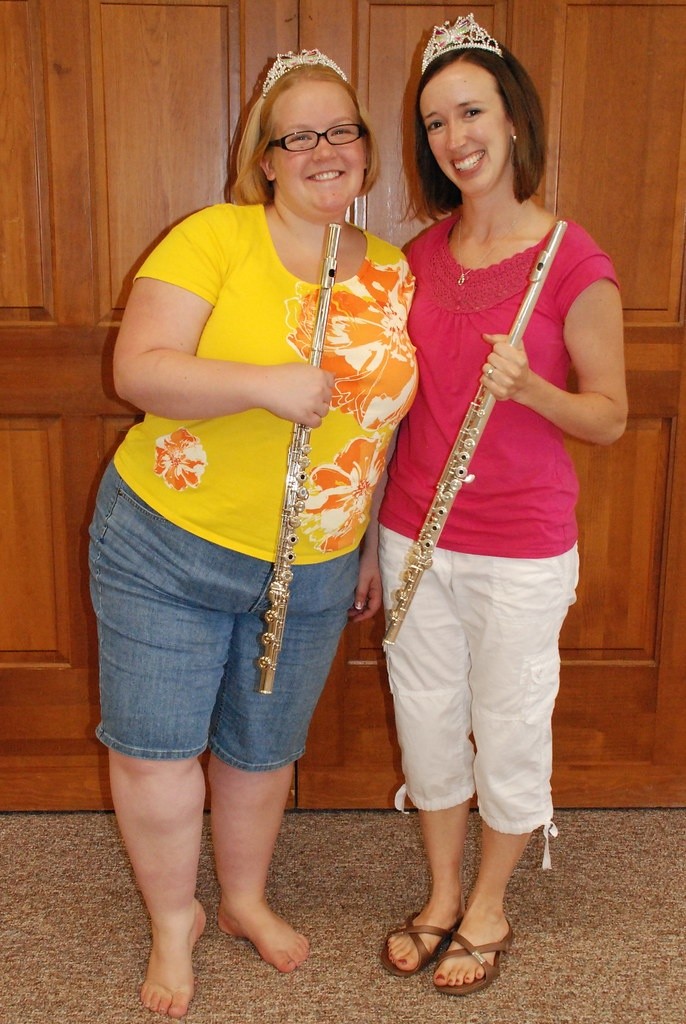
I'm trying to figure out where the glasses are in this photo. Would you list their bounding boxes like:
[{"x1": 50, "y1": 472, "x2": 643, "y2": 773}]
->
[{"x1": 268, "y1": 124, "x2": 368, "y2": 152}]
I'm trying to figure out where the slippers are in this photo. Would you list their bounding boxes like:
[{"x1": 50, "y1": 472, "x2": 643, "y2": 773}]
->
[
  {"x1": 382, "y1": 913, "x2": 464, "y2": 976},
  {"x1": 434, "y1": 917, "x2": 515, "y2": 995}
]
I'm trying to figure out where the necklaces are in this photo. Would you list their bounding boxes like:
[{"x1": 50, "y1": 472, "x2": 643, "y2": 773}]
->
[{"x1": 456, "y1": 198, "x2": 531, "y2": 286}]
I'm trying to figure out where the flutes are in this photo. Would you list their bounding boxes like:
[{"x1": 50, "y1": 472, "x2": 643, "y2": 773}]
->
[
  {"x1": 256, "y1": 222, "x2": 342, "y2": 696},
  {"x1": 382, "y1": 219, "x2": 568, "y2": 651}
]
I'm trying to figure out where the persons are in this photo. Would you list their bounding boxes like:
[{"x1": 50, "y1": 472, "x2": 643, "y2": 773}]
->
[
  {"x1": 84, "y1": 47, "x2": 419, "y2": 1015},
  {"x1": 375, "y1": 11, "x2": 629, "y2": 999}
]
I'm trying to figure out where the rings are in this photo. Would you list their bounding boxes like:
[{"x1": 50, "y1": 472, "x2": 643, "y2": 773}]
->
[{"x1": 486, "y1": 365, "x2": 496, "y2": 378}]
